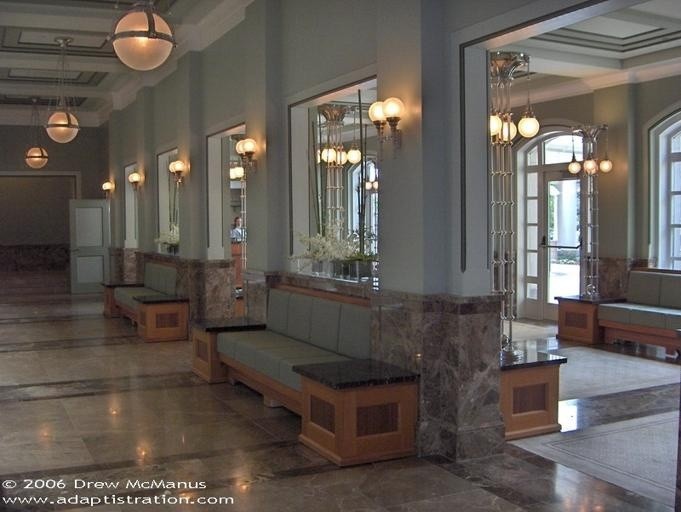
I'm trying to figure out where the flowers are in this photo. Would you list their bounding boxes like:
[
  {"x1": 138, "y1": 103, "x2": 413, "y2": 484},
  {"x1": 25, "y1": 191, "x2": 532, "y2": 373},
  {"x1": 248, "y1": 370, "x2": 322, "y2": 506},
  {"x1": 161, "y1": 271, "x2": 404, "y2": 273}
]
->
[
  {"x1": 288, "y1": 207, "x2": 382, "y2": 267},
  {"x1": 153, "y1": 220, "x2": 180, "y2": 246}
]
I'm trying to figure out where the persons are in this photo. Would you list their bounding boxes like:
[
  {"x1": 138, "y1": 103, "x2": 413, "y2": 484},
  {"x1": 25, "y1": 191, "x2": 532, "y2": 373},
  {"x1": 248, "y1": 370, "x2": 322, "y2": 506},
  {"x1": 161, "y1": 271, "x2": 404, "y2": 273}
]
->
[{"x1": 230, "y1": 217, "x2": 246, "y2": 242}]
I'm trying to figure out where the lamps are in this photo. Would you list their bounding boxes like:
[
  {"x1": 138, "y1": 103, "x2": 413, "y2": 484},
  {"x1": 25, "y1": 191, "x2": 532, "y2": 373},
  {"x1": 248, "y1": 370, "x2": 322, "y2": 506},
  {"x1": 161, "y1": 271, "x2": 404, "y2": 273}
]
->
[{"x1": 23, "y1": 0, "x2": 621, "y2": 198}]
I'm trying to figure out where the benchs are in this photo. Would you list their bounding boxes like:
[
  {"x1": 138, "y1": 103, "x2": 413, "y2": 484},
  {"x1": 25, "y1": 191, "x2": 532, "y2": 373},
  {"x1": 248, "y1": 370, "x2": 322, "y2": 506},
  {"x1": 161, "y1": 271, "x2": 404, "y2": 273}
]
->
[
  {"x1": 214, "y1": 283, "x2": 370, "y2": 415},
  {"x1": 595, "y1": 267, "x2": 680, "y2": 359},
  {"x1": 111, "y1": 262, "x2": 177, "y2": 324}
]
